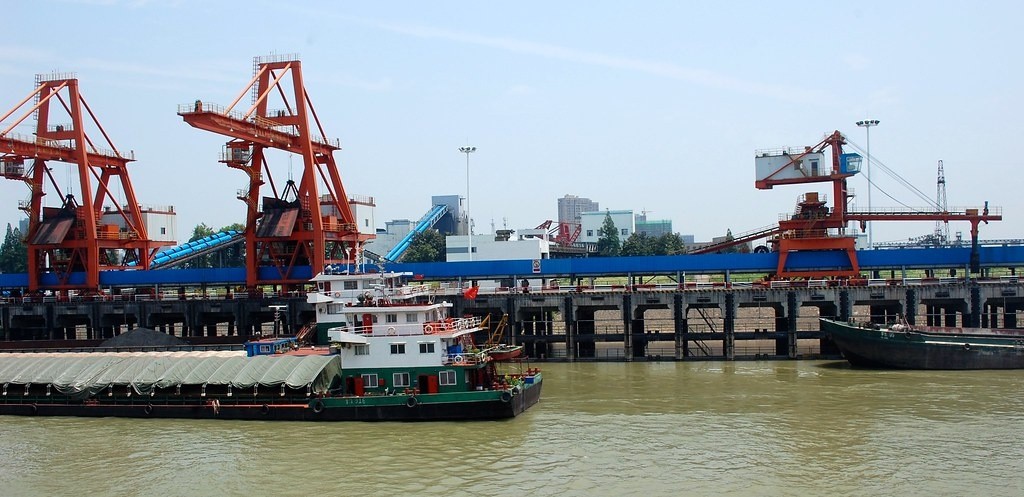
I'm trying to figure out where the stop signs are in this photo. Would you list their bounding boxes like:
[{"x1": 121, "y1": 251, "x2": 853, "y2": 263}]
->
[{"x1": 532, "y1": 259, "x2": 541, "y2": 269}]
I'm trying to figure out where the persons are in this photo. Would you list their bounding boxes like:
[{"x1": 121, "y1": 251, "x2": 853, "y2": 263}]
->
[
  {"x1": 205, "y1": 398, "x2": 219, "y2": 405},
  {"x1": 310, "y1": 390, "x2": 331, "y2": 397}
]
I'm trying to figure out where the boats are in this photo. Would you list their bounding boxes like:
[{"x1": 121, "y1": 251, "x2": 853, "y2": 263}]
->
[
  {"x1": 0, "y1": 232, "x2": 543, "y2": 422},
  {"x1": 475, "y1": 313, "x2": 524, "y2": 361},
  {"x1": 817, "y1": 312, "x2": 1024, "y2": 370}
]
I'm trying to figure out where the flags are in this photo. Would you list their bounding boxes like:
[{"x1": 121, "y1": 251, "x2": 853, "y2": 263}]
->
[{"x1": 464, "y1": 285, "x2": 480, "y2": 298}]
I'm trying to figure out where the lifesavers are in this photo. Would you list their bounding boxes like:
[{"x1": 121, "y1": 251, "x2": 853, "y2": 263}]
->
[
  {"x1": 904, "y1": 332, "x2": 912, "y2": 340},
  {"x1": 500, "y1": 392, "x2": 512, "y2": 403},
  {"x1": 30, "y1": 406, "x2": 36, "y2": 413},
  {"x1": 455, "y1": 356, "x2": 463, "y2": 364},
  {"x1": 425, "y1": 326, "x2": 433, "y2": 334},
  {"x1": 482, "y1": 354, "x2": 486, "y2": 362},
  {"x1": 261, "y1": 406, "x2": 269, "y2": 415},
  {"x1": 313, "y1": 401, "x2": 324, "y2": 414},
  {"x1": 964, "y1": 343, "x2": 971, "y2": 351},
  {"x1": 365, "y1": 291, "x2": 371, "y2": 295},
  {"x1": 407, "y1": 397, "x2": 418, "y2": 408},
  {"x1": 335, "y1": 292, "x2": 341, "y2": 298},
  {"x1": 396, "y1": 290, "x2": 402, "y2": 296},
  {"x1": 144, "y1": 405, "x2": 152, "y2": 415},
  {"x1": 387, "y1": 327, "x2": 395, "y2": 335}
]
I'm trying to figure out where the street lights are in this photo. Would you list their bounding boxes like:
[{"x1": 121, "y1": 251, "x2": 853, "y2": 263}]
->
[
  {"x1": 458, "y1": 146, "x2": 477, "y2": 260},
  {"x1": 856, "y1": 120, "x2": 881, "y2": 251}
]
